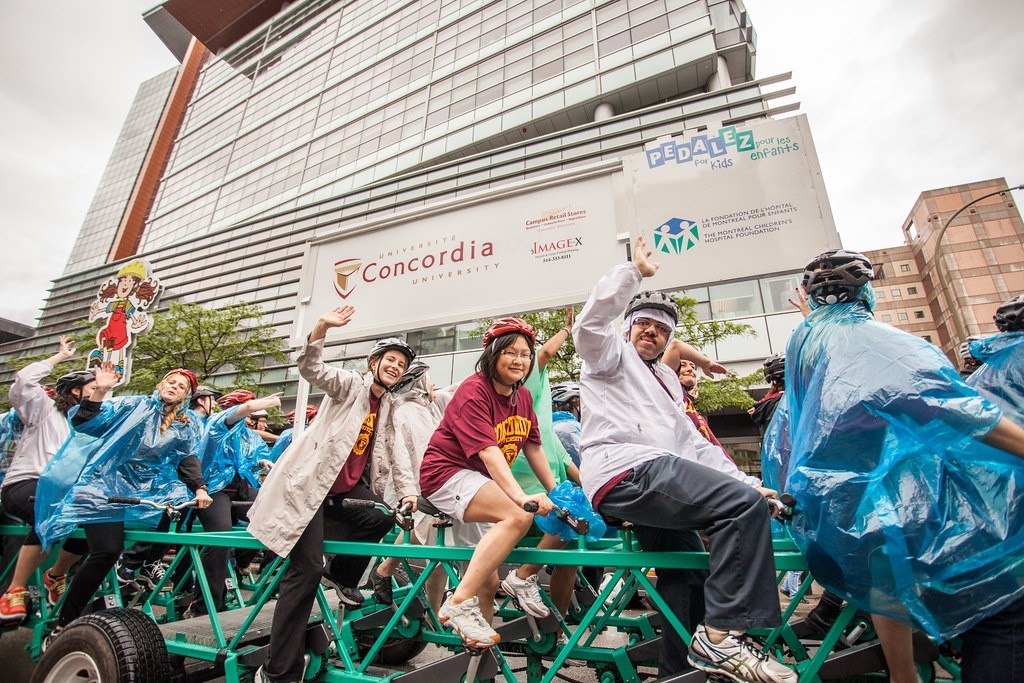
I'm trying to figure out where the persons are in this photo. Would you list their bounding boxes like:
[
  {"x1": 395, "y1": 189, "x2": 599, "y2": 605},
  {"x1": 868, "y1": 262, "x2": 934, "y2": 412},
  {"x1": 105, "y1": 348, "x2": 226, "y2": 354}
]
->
[
  {"x1": 39, "y1": 359, "x2": 214, "y2": 653},
  {"x1": 371, "y1": 307, "x2": 616, "y2": 669},
  {"x1": 114, "y1": 381, "x2": 320, "y2": 618},
  {"x1": 0, "y1": 335, "x2": 96, "y2": 620},
  {"x1": 246, "y1": 304, "x2": 420, "y2": 683},
  {"x1": 567, "y1": 235, "x2": 1024, "y2": 683}
]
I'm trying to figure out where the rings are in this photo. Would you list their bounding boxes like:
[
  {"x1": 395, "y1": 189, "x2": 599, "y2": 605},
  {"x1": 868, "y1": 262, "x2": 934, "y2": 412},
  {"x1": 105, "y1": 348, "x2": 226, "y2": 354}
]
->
[{"x1": 206, "y1": 505, "x2": 209, "y2": 507}]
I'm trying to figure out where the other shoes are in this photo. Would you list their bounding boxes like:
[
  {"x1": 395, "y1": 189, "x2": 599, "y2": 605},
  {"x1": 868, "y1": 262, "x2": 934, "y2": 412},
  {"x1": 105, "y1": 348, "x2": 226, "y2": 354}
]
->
[
  {"x1": 254, "y1": 667, "x2": 272, "y2": 683},
  {"x1": 41, "y1": 629, "x2": 57, "y2": 653},
  {"x1": 779, "y1": 589, "x2": 790, "y2": 596}
]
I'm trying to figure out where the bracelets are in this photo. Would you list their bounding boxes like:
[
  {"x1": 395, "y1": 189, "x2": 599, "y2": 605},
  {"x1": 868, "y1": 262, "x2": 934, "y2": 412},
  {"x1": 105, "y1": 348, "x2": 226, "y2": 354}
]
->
[
  {"x1": 200, "y1": 485, "x2": 208, "y2": 493},
  {"x1": 562, "y1": 328, "x2": 570, "y2": 337}
]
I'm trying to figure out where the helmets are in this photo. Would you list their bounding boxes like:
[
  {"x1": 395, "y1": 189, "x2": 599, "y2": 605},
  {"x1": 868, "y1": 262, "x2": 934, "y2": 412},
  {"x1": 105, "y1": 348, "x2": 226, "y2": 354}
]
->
[
  {"x1": 549, "y1": 381, "x2": 579, "y2": 407},
  {"x1": 41, "y1": 385, "x2": 56, "y2": 399},
  {"x1": 396, "y1": 362, "x2": 430, "y2": 385},
  {"x1": 216, "y1": 388, "x2": 255, "y2": 408},
  {"x1": 764, "y1": 352, "x2": 787, "y2": 384},
  {"x1": 801, "y1": 249, "x2": 873, "y2": 305},
  {"x1": 56, "y1": 371, "x2": 95, "y2": 395},
  {"x1": 164, "y1": 368, "x2": 198, "y2": 395},
  {"x1": 483, "y1": 317, "x2": 535, "y2": 350},
  {"x1": 192, "y1": 385, "x2": 222, "y2": 401},
  {"x1": 959, "y1": 335, "x2": 981, "y2": 358},
  {"x1": 287, "y1": 405, "x2": 319, "y2": 422},
  {"x1": 993, "y1": 295, "x2": 1023, "y2": 333},
  {"x1": 367, "y1": 337, "x2": 416, "y2": 371},
  {"x1": 248, "y1": 409, "x2": 269, "y2": 418},
  {"x1": 624, "y1": 290, "x2": 678, "y2": 327}
]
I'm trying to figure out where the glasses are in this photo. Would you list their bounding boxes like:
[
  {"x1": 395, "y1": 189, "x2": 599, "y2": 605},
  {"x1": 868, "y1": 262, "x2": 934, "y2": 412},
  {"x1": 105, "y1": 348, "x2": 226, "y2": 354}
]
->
[{"x1": 501, "y1": 348, "x2": 533, "y2": 363}]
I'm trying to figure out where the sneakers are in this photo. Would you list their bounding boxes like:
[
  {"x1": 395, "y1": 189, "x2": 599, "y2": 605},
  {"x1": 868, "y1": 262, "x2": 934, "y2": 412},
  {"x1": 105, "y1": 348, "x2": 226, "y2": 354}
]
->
[
  {"x1": 687, "y1": 621, "x2": 799, "y2": 683},
  {"x1": 114, "y1": 558, "x2": 550, "y2": 648},
  {"x1": 544, "y1": 642, "x2": 587, "y2": 668},
  {"x1": 1, "y1": 587, "x2": 29, "y2": 621},
  {"x1": 44, "y1": 568, "x2": 66, "y2": 606}
]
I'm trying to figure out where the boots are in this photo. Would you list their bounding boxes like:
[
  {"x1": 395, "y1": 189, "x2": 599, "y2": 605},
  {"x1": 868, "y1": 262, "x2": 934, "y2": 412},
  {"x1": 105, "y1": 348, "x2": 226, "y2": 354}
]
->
[{"x1": 804, "y1": 597, "x2": 853, "y2": 648}]
